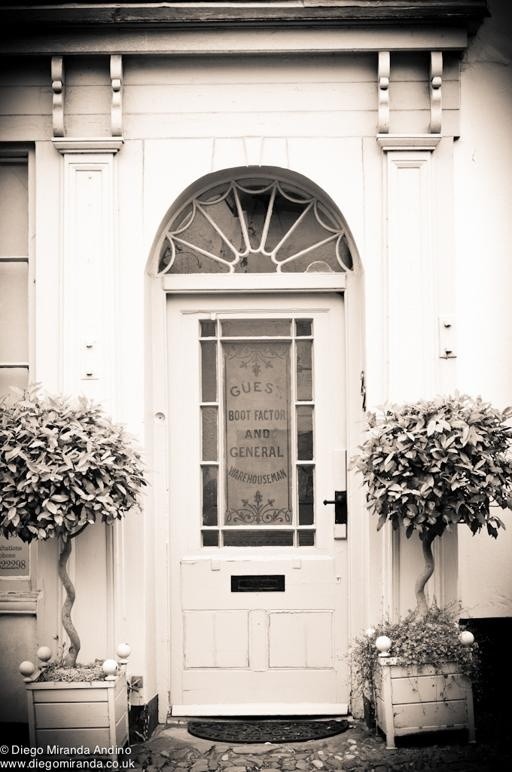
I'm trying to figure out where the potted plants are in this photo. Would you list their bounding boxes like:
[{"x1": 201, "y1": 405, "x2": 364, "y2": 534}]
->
[
  {"x1": 0, "y1": 379, "x2": 148, "y2": 768},
  {"x1": 348, "y1": 386, "x2": 512, "y2": 755}
]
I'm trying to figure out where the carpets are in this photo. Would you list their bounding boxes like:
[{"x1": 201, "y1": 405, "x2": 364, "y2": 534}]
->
[{"x1": 187, "y1": 720, "x2": 352, "y2": 745}]
